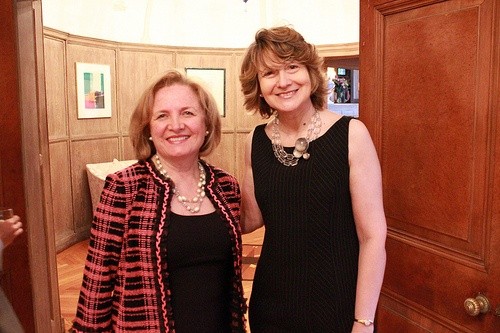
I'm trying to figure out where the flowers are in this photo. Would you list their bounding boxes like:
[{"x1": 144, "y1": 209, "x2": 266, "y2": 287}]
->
[{"x1": 331, "y1": 77, "x2": 354, "y2": 95}]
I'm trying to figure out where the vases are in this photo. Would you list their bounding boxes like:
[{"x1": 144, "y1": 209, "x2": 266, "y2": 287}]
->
[{"x1": 333, "y1": 86, "x2": 350, "y2": 103}]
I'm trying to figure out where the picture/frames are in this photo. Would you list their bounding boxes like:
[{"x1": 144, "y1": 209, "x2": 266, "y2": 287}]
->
[
  {"x1": 185, "y1": 66, "x2": 226, "y2": 118},
  {"x1": 75, "y1": 61, "x2": 112, "y2": 119}
]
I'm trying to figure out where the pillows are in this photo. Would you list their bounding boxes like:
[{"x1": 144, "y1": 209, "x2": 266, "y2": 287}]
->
[{"x1": 106, "y1": 158, "x2": 128, "y2": 174}]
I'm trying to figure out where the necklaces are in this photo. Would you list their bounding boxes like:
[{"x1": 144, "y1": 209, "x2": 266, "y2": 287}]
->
[
  {"x1": 155, "y1": 154, "x2": 208, "y2": 214},
  {"x1": 271, "y1": 111, "x2": 324, "y2": 167}
]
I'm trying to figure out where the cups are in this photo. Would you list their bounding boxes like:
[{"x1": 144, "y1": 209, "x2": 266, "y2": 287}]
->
[{"x1": 0, "y1": 209, "x2": 13, "y2": 220}]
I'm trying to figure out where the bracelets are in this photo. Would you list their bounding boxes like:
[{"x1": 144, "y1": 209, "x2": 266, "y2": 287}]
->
[{"x1": 355, "y1": 318, "x2": 375, "y2": 326}]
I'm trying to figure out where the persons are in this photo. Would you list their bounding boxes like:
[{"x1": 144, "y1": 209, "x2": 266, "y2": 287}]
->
[
  {"x1": 329, "y1": 74, "x2": 349, "y2": 103},
  {"x1": 239, "y1": 27, "x2": 387, "y2": 333},
  {"x1": 0, "y1": 210, "x2": 24, "y2": 333},
  {"x1": 68, "y1": 69, "x2": 248, "y2": 333}
]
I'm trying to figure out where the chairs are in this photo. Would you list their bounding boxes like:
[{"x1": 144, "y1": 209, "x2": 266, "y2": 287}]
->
[{"x1": 86, "y1": 159, "x2": 138, "y2": 215}]
